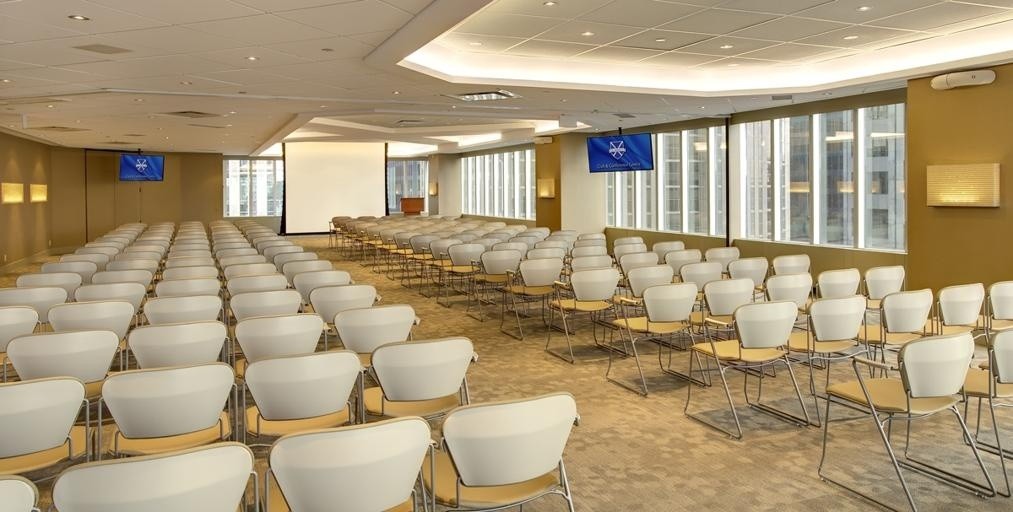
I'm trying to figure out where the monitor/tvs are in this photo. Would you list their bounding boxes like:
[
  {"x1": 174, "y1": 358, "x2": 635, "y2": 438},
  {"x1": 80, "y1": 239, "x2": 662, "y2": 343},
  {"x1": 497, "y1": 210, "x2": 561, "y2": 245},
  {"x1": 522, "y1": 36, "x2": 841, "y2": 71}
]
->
[
  {"x1": 586, "y1": 130, "x2": 655, "y2": 174},
  {"x1": 118, "y1": 154, "x2": 166, "y2": 183}
]
